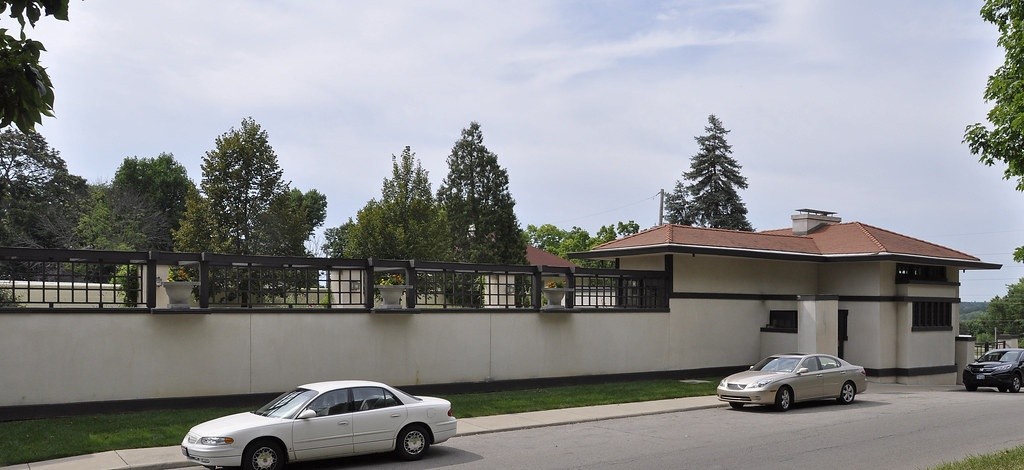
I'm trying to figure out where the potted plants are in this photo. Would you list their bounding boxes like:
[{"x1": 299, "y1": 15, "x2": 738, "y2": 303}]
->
[
  {"x1": 160, "y1": 265, "x2": 201, "y2": 309},
  {"x1": 374, "y1": 275, "x2": 413, "y2": 309},
  {"x1": 540, "y1": 280, "x2": 574, "y2": 309}
]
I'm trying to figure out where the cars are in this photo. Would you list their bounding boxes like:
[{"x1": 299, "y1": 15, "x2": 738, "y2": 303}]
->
[
  {"x1": 962, "y1": 348, "x2": 1024, "y2": 393},
  {"x1": 716, "y1": 352, "x2": 867, "y2": 412},
  {"x1": 180, "y1": 379, "x2": 458, "y2": 470}
]
